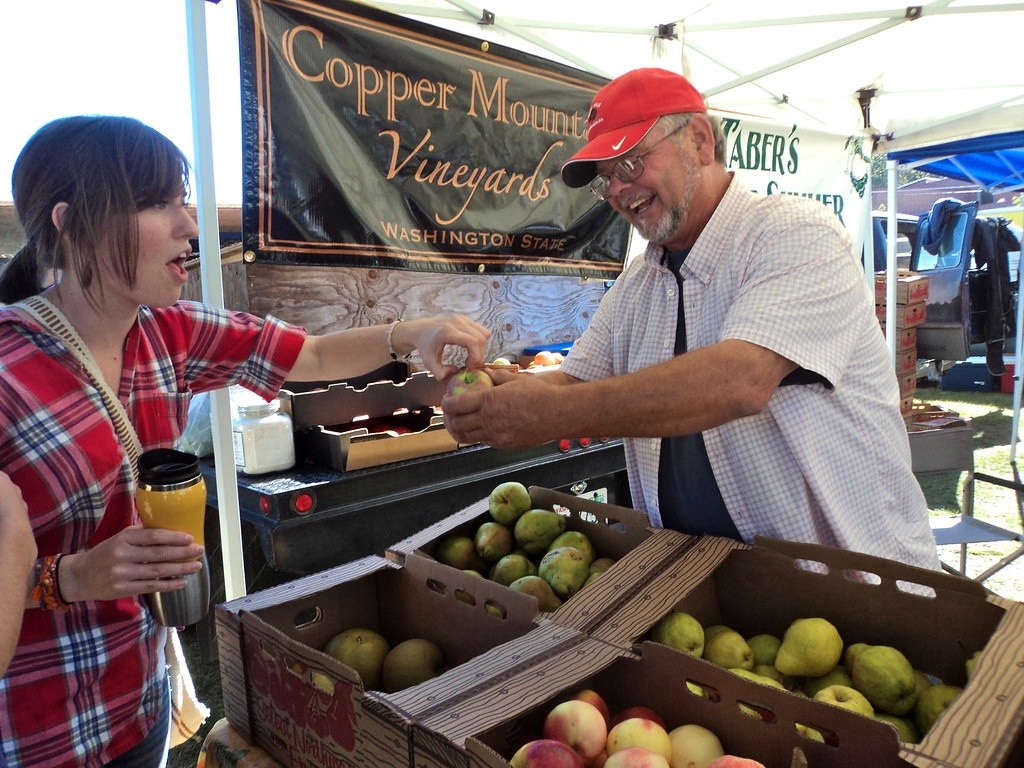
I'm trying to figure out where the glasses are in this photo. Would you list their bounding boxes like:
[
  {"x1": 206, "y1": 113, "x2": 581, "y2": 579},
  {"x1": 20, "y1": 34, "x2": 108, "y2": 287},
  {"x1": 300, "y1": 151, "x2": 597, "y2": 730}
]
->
[{"x1": 586, "y1": 118, "x2": 691, "y2": 202}]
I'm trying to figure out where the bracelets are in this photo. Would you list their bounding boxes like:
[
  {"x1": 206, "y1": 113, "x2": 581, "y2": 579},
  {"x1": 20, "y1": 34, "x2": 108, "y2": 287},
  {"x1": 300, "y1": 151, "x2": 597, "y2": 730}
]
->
[
  {"x1": 33, "y1": 553, "x2": 77, "y2": 613},
  {"x1": 385, "y1": 318, "x2": 414, "y2": 364}
]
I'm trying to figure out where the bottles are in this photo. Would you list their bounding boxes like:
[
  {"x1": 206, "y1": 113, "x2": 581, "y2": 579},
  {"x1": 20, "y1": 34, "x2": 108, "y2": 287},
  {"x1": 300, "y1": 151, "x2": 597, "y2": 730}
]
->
[{"x1": 232, "y1": 400, "x2": 295, "y2": 474}]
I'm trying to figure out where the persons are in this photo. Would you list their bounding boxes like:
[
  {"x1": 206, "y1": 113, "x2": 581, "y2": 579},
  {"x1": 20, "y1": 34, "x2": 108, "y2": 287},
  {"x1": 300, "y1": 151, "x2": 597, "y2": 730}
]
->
[
  {"x1": 0, "y1": 471, "x2": 39, "y2": 673},
  {"x1": 0, "y1": 116, "x2": 490, "y2": 768},
  {"x1": 440, "y1": 66, "x2": 943, "y2": 601}
]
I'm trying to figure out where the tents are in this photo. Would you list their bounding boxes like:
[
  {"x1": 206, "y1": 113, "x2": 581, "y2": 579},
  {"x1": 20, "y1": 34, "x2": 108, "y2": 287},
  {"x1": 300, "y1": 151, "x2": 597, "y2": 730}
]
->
[{"x1": 885, "y1": 130, "x2": 1024, "y2": 373}]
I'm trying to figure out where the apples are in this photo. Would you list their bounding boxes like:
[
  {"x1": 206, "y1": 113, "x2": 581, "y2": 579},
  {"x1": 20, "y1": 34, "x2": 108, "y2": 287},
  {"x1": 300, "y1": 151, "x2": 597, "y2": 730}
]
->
[
  {"x1": 505, "y1": 688, "x2": 766, "y2": 768},
  {"x1": 343, "y1": 423, "x2": 411, "y2": 435},
  {"x1": 447, "y1": 368, "x2": 494, "y2": 398}
]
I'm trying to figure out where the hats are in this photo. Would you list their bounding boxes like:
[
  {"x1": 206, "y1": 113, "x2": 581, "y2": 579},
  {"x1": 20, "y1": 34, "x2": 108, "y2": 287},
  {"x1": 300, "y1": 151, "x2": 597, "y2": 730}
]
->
[{"x1": 561, "y1": 67, "x2": 707, "y2": 188}]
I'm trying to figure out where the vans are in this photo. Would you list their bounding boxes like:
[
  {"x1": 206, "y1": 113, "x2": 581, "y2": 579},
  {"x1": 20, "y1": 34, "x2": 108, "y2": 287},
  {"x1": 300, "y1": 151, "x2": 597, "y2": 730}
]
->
[{"x1": 873, "y1": 201, "x2": 1022, "y2": 361}]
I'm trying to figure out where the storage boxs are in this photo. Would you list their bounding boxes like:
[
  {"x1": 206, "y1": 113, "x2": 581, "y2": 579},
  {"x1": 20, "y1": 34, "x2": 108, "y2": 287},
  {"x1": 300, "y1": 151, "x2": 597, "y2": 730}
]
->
[
  {"x1": 898, "y1": 378, "x2": 917, "y2": 399},
  {"x1": 875, "y1": 304, "x2": 929, "y2": 329},
  {"x1": 893, "y1": 347, "x2": 919, "y2": 375},
  {"x1": 871, "y1": 271, "x2": 928, "y2": 303},
  {"x1": 900, "y1": 402, "x2": 911, "y2": 414},
  {"x1": 881, "y1": 330, "x2": 919, "y2": 351},
  {"x1": 217, "y1": 484, "x2": 1023, "y2": 767},
  {"x1": 269, "y1": 367, "x2": 473, "y2": 475}
]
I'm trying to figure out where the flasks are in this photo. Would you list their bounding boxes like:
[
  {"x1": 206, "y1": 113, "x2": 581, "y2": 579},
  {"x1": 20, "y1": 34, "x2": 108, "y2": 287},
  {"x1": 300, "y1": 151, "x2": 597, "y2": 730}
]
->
[{"x1": 136, "y1": 448, "x2": 210, "y2": 628}]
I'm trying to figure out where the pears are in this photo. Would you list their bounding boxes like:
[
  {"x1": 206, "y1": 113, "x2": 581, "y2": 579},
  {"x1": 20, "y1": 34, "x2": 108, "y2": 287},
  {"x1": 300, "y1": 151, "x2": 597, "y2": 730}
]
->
[
  {"x1": 433, "y1": 481, "x2": 614, "y2": 617},
  {"x1": 308, "y1": 631, "x2": 443, "y2": 693},
  {"x1": 493, "y1": 350, "x2": 566, "y2": 373},
  {"x1": 649, "y1": 613, "x2": 988, "y2": 745}
]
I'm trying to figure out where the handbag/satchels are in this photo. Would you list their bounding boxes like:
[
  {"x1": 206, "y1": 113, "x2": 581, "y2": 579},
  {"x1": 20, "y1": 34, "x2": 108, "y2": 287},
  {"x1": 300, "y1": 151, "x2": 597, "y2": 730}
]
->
[{"x1": 165, "y1": 626, "x2": 210, "y2": 752}]
